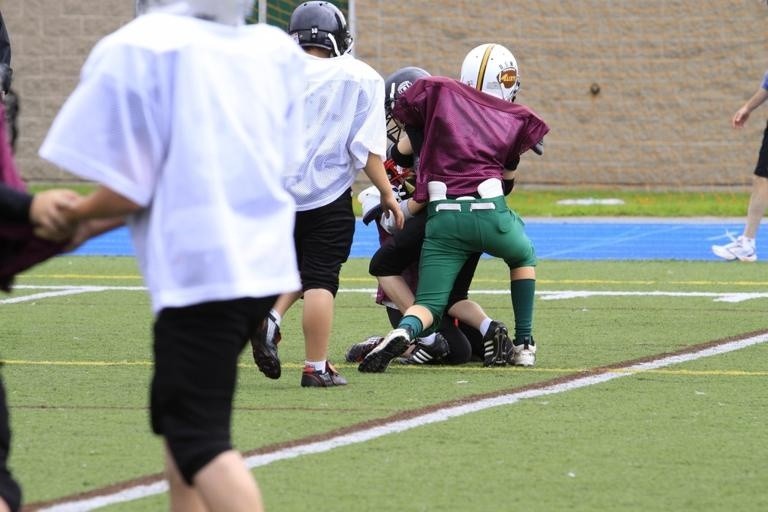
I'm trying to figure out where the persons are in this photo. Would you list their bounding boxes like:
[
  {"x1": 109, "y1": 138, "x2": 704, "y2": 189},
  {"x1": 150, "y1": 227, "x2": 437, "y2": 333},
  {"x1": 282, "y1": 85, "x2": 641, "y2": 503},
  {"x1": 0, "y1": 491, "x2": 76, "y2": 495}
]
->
[
  {"x1": 0, "y1": 12, "x2": 83, "y2": 512},
  {"x1": 249, "y1": 0, "x2": 405, "y2": 387},
  {"x1": 32, "y1": 0, "x2": 304, "y2": 512},
  {"x1": 712, "y1": 75, "x2": 768, "y2": 263},
  {"x1": 345, "y1": 43, "x2": 551, "y2": 372}
]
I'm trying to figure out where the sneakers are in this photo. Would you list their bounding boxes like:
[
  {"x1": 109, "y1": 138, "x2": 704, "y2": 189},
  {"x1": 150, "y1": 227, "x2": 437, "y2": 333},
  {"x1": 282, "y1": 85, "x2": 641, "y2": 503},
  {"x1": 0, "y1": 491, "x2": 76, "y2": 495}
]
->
[
  {"x1": 250, "y1": 311, "x2": 281, "y2": 379},
  {"x1": 392, "y1": 333, "x2": 452, "y2": 366},
  {"x1": 482, "y1": 320, "x2": 508, "y2": 368},
  {"x1": 711, "y1": 230, "x2": 756, "y2": 263},
  {"x1": 358, "y1": 328, "x2": 411, "y2": 373},
  {"x1": 510, "y1": 336, "x2": 537, "y2": 367},
  {"x1": 344, "y1": 334, "x2": 384, "y2": 363},
  {"x1": 301, "y1": 360, "x2": 348, "y2": 387}
]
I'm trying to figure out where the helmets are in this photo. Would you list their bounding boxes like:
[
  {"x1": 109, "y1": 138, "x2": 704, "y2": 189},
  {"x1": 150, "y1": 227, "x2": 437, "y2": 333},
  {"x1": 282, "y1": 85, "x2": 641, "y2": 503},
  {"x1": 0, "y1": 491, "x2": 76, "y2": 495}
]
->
[
  {"x1": 460, "y1": 43, "x2": 520, "y2": 103},
  {"x1": 385, "y1": 66, "x2": 432, "y2": 144},
  {"x1": 288, "y1": 1, "x2": 354, "y2": 57},
  {"x1": 137, "y1": 0, "x2": 256, "y2": 27}
]
